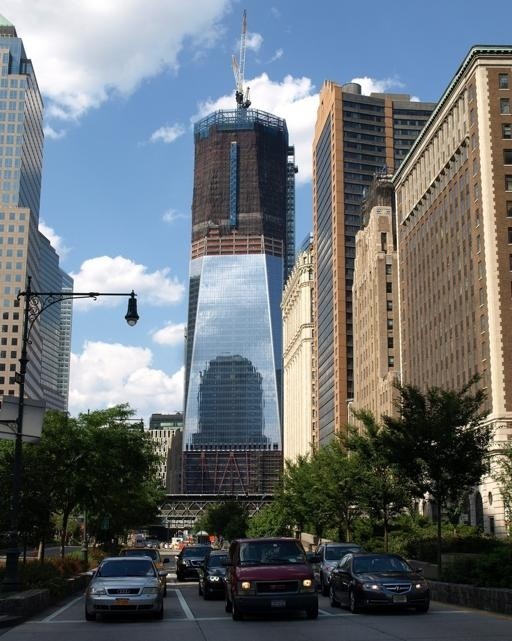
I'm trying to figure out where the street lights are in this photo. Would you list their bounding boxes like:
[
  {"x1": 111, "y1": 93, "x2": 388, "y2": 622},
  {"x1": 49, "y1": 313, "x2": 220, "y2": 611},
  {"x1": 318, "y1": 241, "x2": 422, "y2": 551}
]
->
[{"x1": 3, "y1": 271, "x2": 142, "y2": 592}]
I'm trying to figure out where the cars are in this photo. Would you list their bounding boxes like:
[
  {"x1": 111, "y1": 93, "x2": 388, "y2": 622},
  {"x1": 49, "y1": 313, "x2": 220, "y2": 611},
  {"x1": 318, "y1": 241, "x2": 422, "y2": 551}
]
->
[
  {"x1": 119, "y1": 544, "x2": 395, "y2": 599},
  {"x1": 330, "y1": 551, "x2": 432, "y2": 617},
  {"x1": 86, "y1": 554, "x2": 169, "y2": 620}
]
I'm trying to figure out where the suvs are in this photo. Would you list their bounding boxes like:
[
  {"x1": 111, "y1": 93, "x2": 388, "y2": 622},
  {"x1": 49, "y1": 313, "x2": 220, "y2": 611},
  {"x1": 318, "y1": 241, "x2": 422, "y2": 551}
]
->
[{"x1": 224, "y1": 537, "x2": 320, "y2": 624}]
